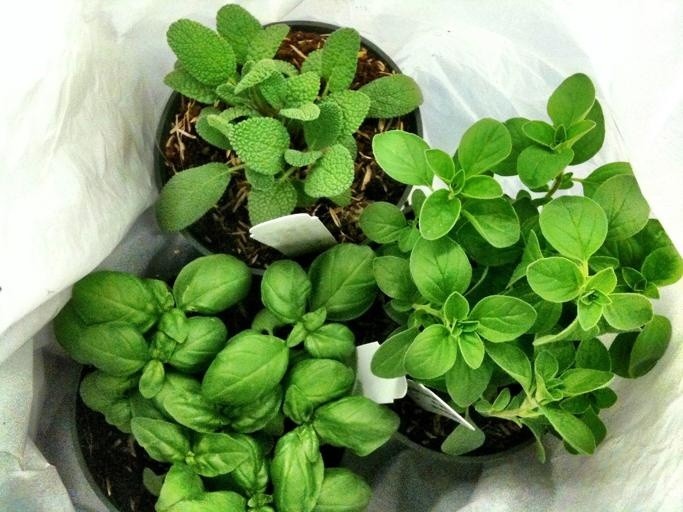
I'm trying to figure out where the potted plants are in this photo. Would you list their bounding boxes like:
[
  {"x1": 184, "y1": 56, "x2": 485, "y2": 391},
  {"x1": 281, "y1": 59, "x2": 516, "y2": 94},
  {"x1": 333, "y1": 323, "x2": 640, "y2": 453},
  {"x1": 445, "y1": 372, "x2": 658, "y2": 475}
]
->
[
  {"x1": 155, "y1": 3, "x2": 424, "y2": 277},
  {"x1": 53, "y1": 242, "x2": 401, "y2": 512},
  {"x1": 361, "y1": 74, "x2": 683, "y2": 482}
]
